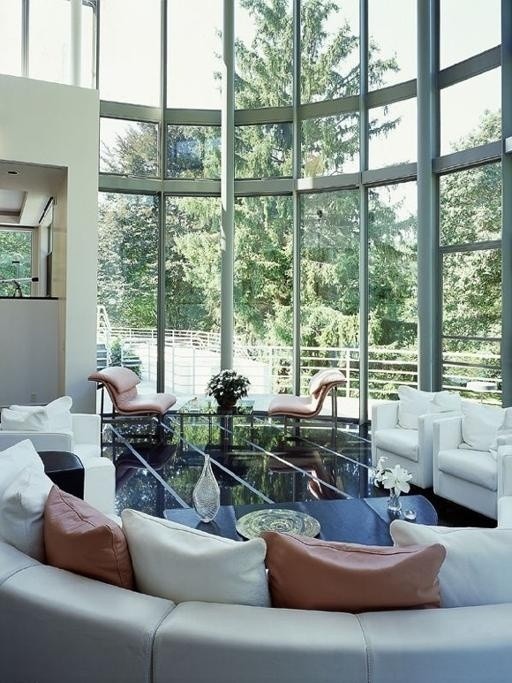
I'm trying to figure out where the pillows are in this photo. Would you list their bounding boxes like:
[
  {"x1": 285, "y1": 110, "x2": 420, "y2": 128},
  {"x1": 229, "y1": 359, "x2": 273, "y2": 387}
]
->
[
  {"x1": 390, "y1": 521, "x2": 510, "y2": 608},
  {"x1": 460, "y1": 406, "x2": 512, "y2": 457},
  {"x1": 1, "y1": 439, "x2": 45, "y2": 490},
  {"x1": 2, "y1": 463, "x2": 52, "y2": 558},
  {"x1": 43, "y1": 484, "x2": 132, "y2": 592},
  {"x1": 1, "y1": 396, "x2": 73, "y2": 430},
  {"x1": 259, "y1": 530, "x2": 446, "y2": 612},
  {"x1": 119, "y1": 508, "x2": 270, "y2": 609},
  {"x1": 395, "y1": 384, "x2": 460, "y2": 431}
]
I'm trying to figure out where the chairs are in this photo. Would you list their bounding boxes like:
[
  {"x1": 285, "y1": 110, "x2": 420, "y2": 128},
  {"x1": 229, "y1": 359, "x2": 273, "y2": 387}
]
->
[
  {"x1": 433, "y1": 407, "x2": 512, "y2": 521},
  {"x1": 268, "y1": 368, "x2": 347, "y2": 451},
  {"x1": 371, "y1": 396, "x2": 478, "y2": 489},
  {"x1": 0, "y1": 414, "x2": 102, "y2": 456},
  {"x1": 88, "y1": 366, "x2": 176, "y2": 447}
]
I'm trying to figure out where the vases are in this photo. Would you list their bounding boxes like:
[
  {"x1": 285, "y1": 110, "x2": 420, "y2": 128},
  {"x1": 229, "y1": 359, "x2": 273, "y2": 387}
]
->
[
  {"x1": 193, "y1": 455, "x2": 221, "y2": 524},
  {"x1": 387, "y1": 490, "x2": 402, "y2": 517},
  {"x1": 216, "y1": 395, "x2": 237, "y2": 407}
]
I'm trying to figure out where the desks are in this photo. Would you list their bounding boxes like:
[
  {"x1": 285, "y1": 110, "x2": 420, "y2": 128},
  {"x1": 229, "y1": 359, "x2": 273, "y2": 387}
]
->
[{"x1": 161, "y1": 495, "x2": 437, "y2": 550}]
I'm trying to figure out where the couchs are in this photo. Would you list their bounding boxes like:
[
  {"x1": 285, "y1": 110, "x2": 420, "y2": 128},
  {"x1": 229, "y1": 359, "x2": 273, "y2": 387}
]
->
[{"x1": 0, "y1": 456, "x2": 512, "y2": 683}]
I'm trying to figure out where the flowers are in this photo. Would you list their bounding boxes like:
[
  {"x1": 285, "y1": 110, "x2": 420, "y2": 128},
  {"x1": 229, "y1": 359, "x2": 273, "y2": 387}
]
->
[
  {"x1": 372, "y1": 459, "x2": 412, "y2": 492},
  {"x1": 206, "y1": 369, "x2": 251, "y2": 399}
]
